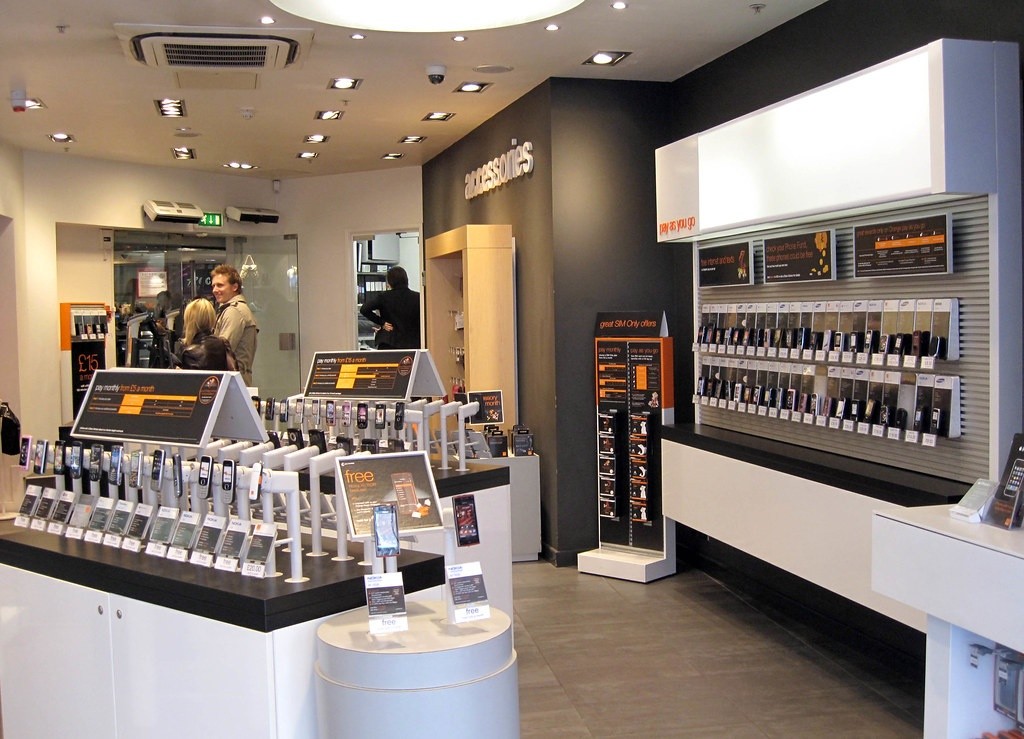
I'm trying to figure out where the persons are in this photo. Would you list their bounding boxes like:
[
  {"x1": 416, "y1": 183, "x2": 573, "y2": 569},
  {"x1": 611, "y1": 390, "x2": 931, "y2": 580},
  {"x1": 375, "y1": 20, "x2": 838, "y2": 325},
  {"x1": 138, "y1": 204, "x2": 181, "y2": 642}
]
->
[
  {"x1": 210, "y1": 265, "x2": 259, "y2": 387},
  {"x1": 157, "y1": 291, "x2": 176, "y2": 318},
  {"x1": 361, "y1": 266, "x2": 420, "y2": 350},
  {"x1": 171, "y1": 298, "x2": 239, "y2": 371}
]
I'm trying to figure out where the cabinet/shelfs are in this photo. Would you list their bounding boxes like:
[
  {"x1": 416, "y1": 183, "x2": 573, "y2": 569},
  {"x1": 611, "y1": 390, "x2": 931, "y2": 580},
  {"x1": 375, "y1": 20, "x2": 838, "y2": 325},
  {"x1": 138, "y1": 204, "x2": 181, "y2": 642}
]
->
[
  {"x1": 358, "y1": 270, "x2": 391, "y2": 340},
  {"x1": 0, "y1": 562, "x2": 317, "y2": 739}
]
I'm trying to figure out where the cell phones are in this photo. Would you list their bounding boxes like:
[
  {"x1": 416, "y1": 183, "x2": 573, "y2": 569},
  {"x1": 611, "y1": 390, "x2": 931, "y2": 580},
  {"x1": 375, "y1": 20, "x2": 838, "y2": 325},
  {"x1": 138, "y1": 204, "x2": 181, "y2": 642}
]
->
[
  {"x1": 77, "y1": 322, "x2": 101, "y2": 335},
  {"x1": 372, "y1": 503, "x2": 401, "y2": 558},
  {"x1": 17, "y1": 395, "x2": 406, "y2": 506},
  {"x1": 451, "y1": 493, "x2": 481, "y2": 548},
  {"x1": 694, "y1": 324, "x2": 942, "y2": 436}
]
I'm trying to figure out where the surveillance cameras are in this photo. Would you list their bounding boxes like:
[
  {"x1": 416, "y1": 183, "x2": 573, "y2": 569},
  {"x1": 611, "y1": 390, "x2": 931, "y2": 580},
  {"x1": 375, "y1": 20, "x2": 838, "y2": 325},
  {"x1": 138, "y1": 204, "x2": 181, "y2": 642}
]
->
[{"x1": 425, "y1": 63, "x2": 448, "y2": 86}]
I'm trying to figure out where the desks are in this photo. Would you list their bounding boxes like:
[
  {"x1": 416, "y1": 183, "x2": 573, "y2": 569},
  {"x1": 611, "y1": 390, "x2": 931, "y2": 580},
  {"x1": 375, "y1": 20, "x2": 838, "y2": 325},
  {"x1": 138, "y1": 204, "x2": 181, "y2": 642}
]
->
[
  {"x1": 873, "y1": 506, "x2": 1024, "y2": 739},
  {"x1": 316, "y1": 602, "x2": 521, "y2": 739},
  {"x1": 453, "y1": 449, "x2": 542, "y2": 562}
]
